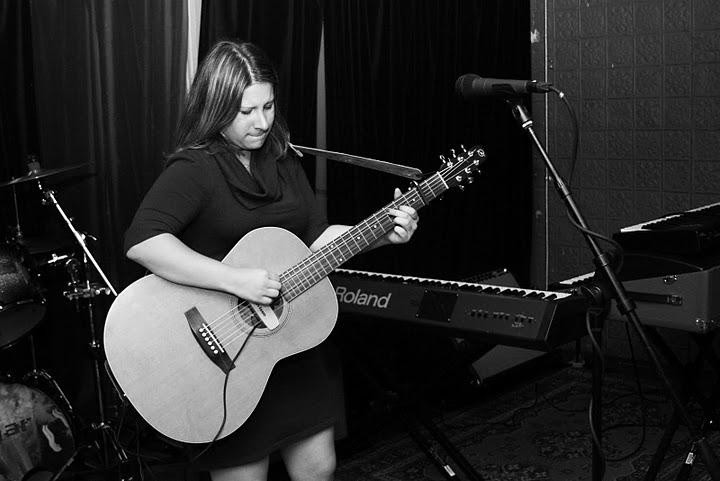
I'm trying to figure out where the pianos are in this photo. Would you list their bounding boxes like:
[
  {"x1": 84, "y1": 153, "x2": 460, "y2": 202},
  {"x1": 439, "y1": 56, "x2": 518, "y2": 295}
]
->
[
  {"x1": 324, "y1": 267, "x2": 584, "y2": 354},
  {"x1": 548, "y1": 201, "x2": 720, "y2": 335}
]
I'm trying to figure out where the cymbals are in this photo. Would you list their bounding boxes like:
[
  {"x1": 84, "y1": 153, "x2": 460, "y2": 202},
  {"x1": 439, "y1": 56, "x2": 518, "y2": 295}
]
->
[{"x1": 4, "y1": 164, "x2": 83, "y2": 186}]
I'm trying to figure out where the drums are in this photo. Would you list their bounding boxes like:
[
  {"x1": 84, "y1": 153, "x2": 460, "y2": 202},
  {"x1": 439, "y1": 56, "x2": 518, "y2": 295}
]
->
[
  {"x1": 0, "y1": 365, "x2": 75, "y2": 481},
  {"x1": 0, "y1": 246, "x2": 57, "y2": 366}
]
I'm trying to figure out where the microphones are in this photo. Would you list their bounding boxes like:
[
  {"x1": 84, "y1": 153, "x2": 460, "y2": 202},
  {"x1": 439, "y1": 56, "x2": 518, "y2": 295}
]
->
[{"x1": 456, "y1": 70, "x2": 533, "y2": 94}]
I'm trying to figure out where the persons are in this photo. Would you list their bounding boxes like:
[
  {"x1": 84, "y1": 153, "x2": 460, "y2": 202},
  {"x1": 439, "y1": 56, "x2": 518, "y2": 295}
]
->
[{"x1": 121, "y1": 40, "x2": 420, "y2": 480}]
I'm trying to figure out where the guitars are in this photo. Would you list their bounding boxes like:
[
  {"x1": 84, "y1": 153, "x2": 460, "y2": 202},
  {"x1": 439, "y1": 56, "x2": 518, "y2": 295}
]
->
[{"x1": 100, "y1": 144, "x2": 487, "y2": 451}]
